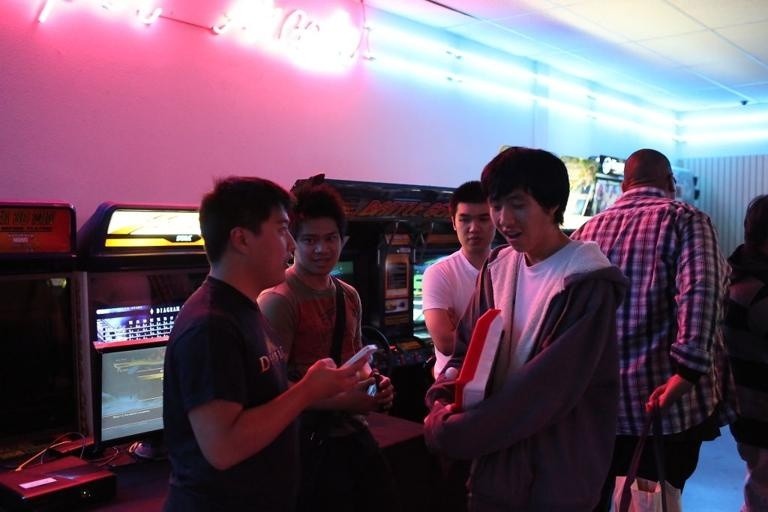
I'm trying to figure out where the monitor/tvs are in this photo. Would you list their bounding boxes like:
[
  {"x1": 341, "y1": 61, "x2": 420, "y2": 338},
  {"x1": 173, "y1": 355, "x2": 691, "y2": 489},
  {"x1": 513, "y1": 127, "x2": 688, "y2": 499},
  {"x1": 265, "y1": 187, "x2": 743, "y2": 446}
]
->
[
  {"x1": 411, "y1": 254, "x2": 456, "y2": 327},
  {"x1": 94, "y1": 337, "x2": 169, "y2": 450},
  {"x1": 579, "y1": 175, "x2": 624, "y2": 226}
]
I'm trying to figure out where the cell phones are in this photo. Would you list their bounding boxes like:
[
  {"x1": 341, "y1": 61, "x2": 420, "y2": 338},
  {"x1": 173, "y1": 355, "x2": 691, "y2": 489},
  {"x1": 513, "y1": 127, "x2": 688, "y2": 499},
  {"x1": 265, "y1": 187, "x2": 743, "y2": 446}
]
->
[{"x1": 336, "y1": 343, "x2": 378, "y2": 372}]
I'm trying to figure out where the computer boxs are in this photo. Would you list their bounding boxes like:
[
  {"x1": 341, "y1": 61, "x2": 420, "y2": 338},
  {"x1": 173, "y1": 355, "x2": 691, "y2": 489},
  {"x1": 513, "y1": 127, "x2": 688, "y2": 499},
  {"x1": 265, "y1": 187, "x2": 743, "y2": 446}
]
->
[{"x1": 0, "y1": 456, "x2": 119, "y2": 512}]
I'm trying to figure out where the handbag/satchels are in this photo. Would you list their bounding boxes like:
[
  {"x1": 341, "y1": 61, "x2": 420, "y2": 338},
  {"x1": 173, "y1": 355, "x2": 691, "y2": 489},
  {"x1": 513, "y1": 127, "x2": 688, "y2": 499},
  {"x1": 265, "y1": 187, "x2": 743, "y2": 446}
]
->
[
  {"x1": 611, "y1": 405, "x2": 682, "y2": 512},
  {"x1": 296, "y1": 277, "x2": 345, "y2": 510}
]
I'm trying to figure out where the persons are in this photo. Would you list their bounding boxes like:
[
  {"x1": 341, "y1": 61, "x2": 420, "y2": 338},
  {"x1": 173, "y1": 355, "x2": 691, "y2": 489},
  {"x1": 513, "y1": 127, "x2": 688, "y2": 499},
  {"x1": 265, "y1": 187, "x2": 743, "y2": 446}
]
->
[
  {"x1": 421, "y1": 181, "x2": 497, "y2": 380},
  {"x1": 256, "y1": 185, "x2": 395, "y2": 412},
  {"x1": 423, "y1": 146, "x2": 630, "y2": 512},
  {"x1": 163, "y1": 175, "x2": 369, "y2": 512},
  {"x1": 726, "y1": 196, "x2": 768, "y2": 512},
  {"x1": 570, "y1": 147, "x2": 734, "y2": 512}
]
